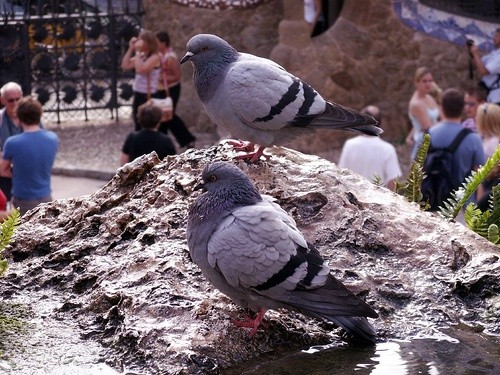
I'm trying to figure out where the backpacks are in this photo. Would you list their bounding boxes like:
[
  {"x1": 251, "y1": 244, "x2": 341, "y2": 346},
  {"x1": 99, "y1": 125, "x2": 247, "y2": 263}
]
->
[{"x1": 421, "y1": 128, "x2": 474, "y2": 212}]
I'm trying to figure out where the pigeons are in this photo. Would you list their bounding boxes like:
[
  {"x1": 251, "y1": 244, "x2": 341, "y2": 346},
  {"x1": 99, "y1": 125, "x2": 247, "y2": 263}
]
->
[
  {"x1": 180, "y1": 33, "x2": 385, "y2": 165},
  {"x1": 187, "y1": 163, "x2": 379, "y2": 348}
]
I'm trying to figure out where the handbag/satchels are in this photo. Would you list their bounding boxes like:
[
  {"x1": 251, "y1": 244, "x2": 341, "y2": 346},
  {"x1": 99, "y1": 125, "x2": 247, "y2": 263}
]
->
[{"x1": 151, "y1": 97, "x2": 173, "y2": 122}]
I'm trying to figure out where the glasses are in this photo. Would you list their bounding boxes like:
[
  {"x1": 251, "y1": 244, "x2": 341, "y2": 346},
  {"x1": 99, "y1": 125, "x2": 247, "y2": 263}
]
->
[
  {"x1": 4, "y1": 97, "x2": 21, "y2": 103},
  {"x1": 464, "y1": 101, "x2": 478, "y2": 106}
]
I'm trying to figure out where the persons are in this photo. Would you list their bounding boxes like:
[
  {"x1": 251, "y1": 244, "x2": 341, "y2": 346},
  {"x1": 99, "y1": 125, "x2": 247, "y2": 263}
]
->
[
  {"x1": 0, "y1": 82, "x2": 60, "y2": 224},
  {"x1": 337, "y1": 28, "x2": 500, "y2": 228},
  {"x1": 120, "y1": 31, "x2": 197, "y2": 166}
]
